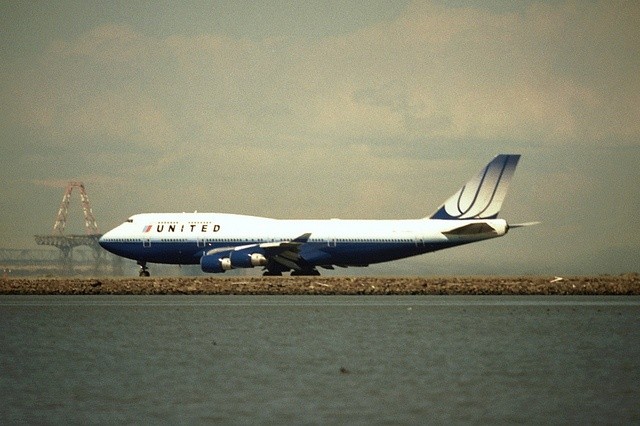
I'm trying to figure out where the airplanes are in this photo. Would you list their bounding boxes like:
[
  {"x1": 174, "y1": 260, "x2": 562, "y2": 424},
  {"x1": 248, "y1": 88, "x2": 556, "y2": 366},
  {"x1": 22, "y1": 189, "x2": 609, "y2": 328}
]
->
[{"x1": 98, "y1": 154, "x2": 542, "y2": 278}]
